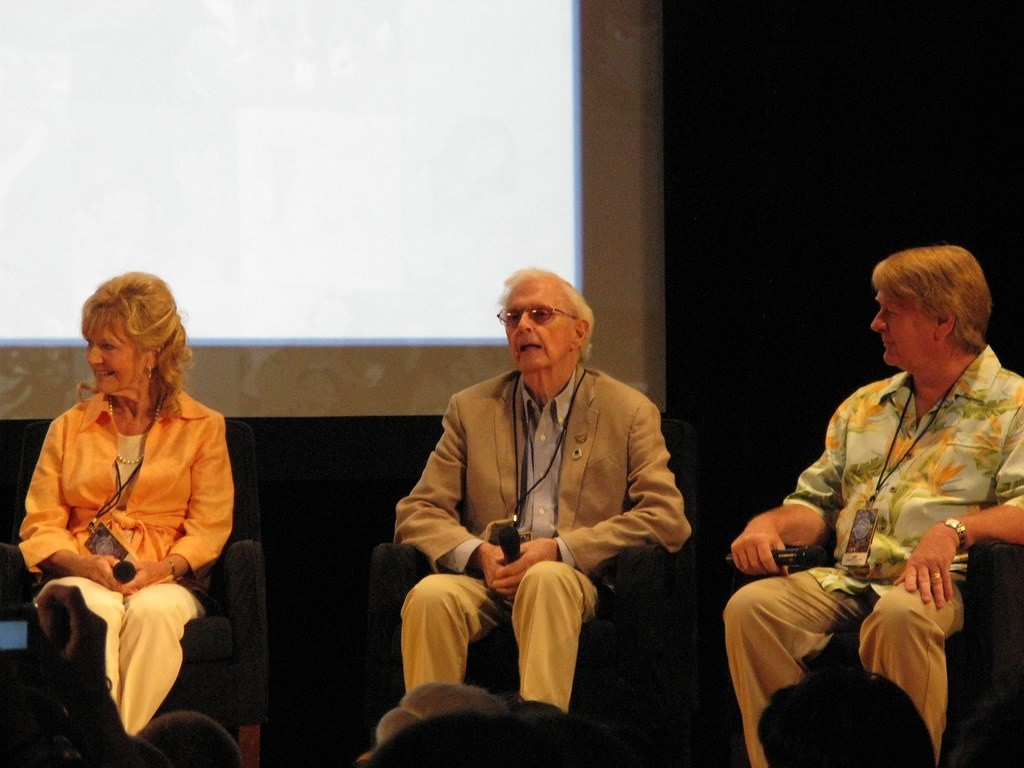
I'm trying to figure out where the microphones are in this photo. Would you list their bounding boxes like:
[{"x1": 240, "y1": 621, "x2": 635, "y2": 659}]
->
[
  {"x1": 724, "y1": 543, "x2": 826, "y2": 569},
  {"x1": 498, "y1": 525, "x2": 522, "y2": 565},
  {"x1": 112, "y1": 560, "x2": 137, "y2": 582}
]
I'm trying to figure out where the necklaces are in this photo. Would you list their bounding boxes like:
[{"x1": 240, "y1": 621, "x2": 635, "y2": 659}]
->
[{"x1": 110, "y1": 391, "x2": 164, "y2": 465}]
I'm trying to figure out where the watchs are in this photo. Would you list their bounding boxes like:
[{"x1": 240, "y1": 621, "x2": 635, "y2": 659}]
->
[{"x1": 942, "y1": 519, "x2": 966, "y2": 550}]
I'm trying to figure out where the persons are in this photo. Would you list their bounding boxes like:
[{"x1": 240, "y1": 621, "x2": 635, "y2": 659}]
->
[
  {"x1": 722, "y1": 246, "x2": 1024, "y2": 768},
  {"x1": 758, "y1": 669, "x2": 936, "y2": 768},
  {"x1": 393, "y1": 269, "x2": 690, "y2": 715},
  {"x1": 18, "y1": 272, "x2": 235, "y2": 736},
  {"x1": 0, "y1": 585, "x2": 642, "y2": 768}
]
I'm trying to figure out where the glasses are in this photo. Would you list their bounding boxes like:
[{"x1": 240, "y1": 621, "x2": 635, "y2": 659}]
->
[{"x1": 497, "y1": 307, "x2": 577, "y2": 323}]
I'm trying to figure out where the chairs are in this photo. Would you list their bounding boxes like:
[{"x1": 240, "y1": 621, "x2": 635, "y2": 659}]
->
[
  {"x1": 13, "y1": 423, "x2": 275, "y2": 768},
  {"x1": 716, "y1": 510, "x2": 1024, "y2": 768},
  {"x1": 366, "y1": 419, "x2": 699, "y2": 768}
]
